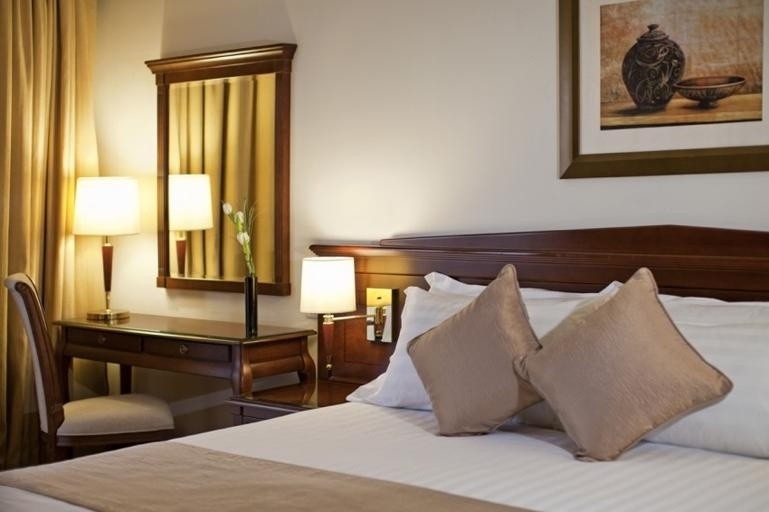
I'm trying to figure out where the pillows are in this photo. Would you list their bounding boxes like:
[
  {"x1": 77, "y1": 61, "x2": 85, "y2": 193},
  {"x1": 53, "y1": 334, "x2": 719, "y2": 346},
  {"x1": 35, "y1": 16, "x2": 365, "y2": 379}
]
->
[
  {"x1": 518, "y1": 270, "x2": 733, "y2": 465},
  {"x1": 579, "y1": 299, "x2": 766, "y2": 461},
  {"x1": 349, "y1": 286, "x2": 606, "y2": 432},
  {"x1": 404, "y1": 268, "x2": 554, "y2": 436}
]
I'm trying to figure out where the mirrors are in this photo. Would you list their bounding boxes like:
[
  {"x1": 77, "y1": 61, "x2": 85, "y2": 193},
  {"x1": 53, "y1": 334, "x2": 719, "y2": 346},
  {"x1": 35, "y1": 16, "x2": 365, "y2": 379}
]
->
[{"x1": 142, "y1": 43, "x2": 297, "y2": 300}]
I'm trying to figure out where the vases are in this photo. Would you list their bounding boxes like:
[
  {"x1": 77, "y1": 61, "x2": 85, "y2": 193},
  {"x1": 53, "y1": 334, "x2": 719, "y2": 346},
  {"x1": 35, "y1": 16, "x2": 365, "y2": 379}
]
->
[{"x1": 244, "y1": 275, "x2": 259, "y2": 339}]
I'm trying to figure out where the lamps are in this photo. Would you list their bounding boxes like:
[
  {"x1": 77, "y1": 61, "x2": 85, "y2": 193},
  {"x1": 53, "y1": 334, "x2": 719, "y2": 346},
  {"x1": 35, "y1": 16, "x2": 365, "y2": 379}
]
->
[
  {"x1": 68, "y1": 174, "x2": 146, "y2": 323},
  {"x1": 165, "y1": 172, "x2": 217, "y2": 279},
  {"x1": 297, "y1": 255, "x2": 387, "y2": 371}
]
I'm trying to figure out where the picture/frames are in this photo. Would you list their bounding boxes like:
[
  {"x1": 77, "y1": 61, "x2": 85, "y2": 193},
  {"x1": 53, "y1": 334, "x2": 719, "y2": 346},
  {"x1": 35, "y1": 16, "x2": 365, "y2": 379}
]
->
[{"x1": 561, "y1": 0, "x2": 768, "y2": 181}]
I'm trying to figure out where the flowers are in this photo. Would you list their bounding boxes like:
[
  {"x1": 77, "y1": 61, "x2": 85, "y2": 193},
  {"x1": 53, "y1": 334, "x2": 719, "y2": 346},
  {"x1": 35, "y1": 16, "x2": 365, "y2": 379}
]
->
[{"x1": 219, "y1": 199, "x2": 259, "y2": 281}]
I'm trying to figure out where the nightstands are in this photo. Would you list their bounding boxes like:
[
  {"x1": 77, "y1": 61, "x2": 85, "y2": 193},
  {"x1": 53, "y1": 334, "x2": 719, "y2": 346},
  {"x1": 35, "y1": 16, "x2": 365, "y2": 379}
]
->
[{"x1": 224, "y1": 378, "x2": 363, "y2": 429}]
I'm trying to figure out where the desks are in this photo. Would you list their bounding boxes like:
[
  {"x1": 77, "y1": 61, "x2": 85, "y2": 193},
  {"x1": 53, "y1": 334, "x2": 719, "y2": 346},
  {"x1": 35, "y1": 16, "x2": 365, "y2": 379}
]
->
[{"x1": 45, "y1": 312, "x2": 316, "y2": 424}]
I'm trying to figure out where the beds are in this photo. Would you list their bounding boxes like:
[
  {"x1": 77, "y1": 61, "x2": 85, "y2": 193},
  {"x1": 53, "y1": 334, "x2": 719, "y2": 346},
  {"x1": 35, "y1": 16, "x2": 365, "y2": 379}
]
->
[{"x1": 1, "y1": 223, "x2": 768, "y2": 511}]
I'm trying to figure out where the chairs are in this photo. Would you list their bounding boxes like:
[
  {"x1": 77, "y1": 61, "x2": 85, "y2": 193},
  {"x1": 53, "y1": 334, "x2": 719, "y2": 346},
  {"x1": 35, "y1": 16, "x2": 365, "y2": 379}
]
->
[{"x1": 1, "y1": 270, "x2": 177, "y2": 461}]
